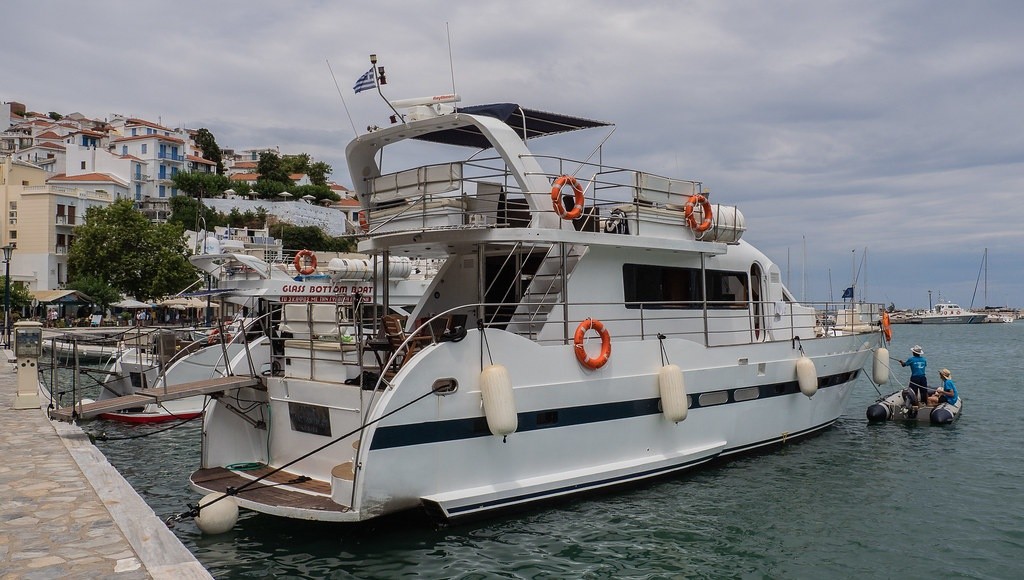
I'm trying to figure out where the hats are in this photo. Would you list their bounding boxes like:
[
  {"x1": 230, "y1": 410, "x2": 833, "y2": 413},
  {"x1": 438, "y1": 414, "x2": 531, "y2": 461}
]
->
[
  {"x1": 910, "y1": 345, "x2": 924, "y2": 354},
  {"x1": 938, "y1": 368, "x2": 951, "y2": 380}
]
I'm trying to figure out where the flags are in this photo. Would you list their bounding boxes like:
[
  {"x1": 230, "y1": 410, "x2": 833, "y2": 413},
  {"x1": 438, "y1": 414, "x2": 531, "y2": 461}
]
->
[{"x1": 351, "y1": 66, "x2": 378, "y2": 94}]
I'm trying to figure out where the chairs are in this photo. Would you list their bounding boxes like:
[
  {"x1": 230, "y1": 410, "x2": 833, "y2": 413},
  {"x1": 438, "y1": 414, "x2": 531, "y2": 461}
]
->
[
  {"x1": 360, "y1": 313, "x2": 408, "y2": 373},
  {"x1": 446, "y1": 314, "x2": 468, "y2": 331},
  {"x1": 419, "y1": 317, "x2": 448, "y2": 345},
  {"x1": 381, "y1": 315, "x2": 432, "y2": 369}
]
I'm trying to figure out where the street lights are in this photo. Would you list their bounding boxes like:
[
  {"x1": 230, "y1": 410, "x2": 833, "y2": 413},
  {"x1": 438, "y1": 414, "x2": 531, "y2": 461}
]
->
[{"x1": 0, "y1": 244, "x2": 18, "y2": 347}]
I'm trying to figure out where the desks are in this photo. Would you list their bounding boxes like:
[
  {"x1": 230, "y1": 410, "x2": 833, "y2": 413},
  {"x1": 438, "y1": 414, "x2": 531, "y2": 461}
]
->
[{"x1": 367, "y1": 338, "x2": 396, "y2": 373}]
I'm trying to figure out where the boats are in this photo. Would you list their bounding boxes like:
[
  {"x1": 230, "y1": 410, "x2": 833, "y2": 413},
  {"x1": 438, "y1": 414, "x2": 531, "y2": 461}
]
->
[
  {"x1": 888, "y1": 303, "x2": 1012, "y2": 325},
  {"x1": 38, "y1": 253, "x2": 308, "y2": 424},
  {"x1": 867, "y1": 387, "x2": 962, "y2": 426},
  {"x1": 186, "y1": 9, "x2": 886, "y2": 524}
]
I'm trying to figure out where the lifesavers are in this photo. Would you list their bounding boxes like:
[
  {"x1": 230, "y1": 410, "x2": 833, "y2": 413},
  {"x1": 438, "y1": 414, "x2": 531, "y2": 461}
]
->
[
  {"x1": 882, "y1": 313, "x2": 892, "y2": 341},
  {"x1": 293, "y1": 249, "x2": 317, "y2": 275},
  {"x1": 359, "y1": 212, "x2": 369, "y2": 230},
  {"x1": 573, "y1": 318, "x2": 613, "y2": 371},
  {"x1": 683, "y1": 194, "x2": 713, "y2": 233},
  {"x1": 208, "y1": 328, "x2": 232, "y2": 344},
  {"x1": 552, "y1": 175, "x2": 586, "y2": 220}
]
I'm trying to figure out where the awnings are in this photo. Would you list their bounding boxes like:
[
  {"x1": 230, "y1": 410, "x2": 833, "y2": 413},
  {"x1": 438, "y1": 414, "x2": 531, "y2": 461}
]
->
[
  {"x1": 412, "y1": 102, "x2": 617, "y2": 150},
  {"x1": 108, "y1": 287, "x2": 260, "y2": 309}
]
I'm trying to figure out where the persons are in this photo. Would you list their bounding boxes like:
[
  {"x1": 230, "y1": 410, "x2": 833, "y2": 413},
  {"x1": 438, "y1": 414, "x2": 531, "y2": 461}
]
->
[
  {"x1": 137, "y1": 310, "x2": 146, "y2": 326},
  {"x1": 41, "y1": 307, "x2": 58, "y2": 328},
  {"x1": 900, "y1": 344, "x2": 928, "y2": 405},
  {"x1": 928, "y1": 368, "x2": 958, "y2": 405}
]
